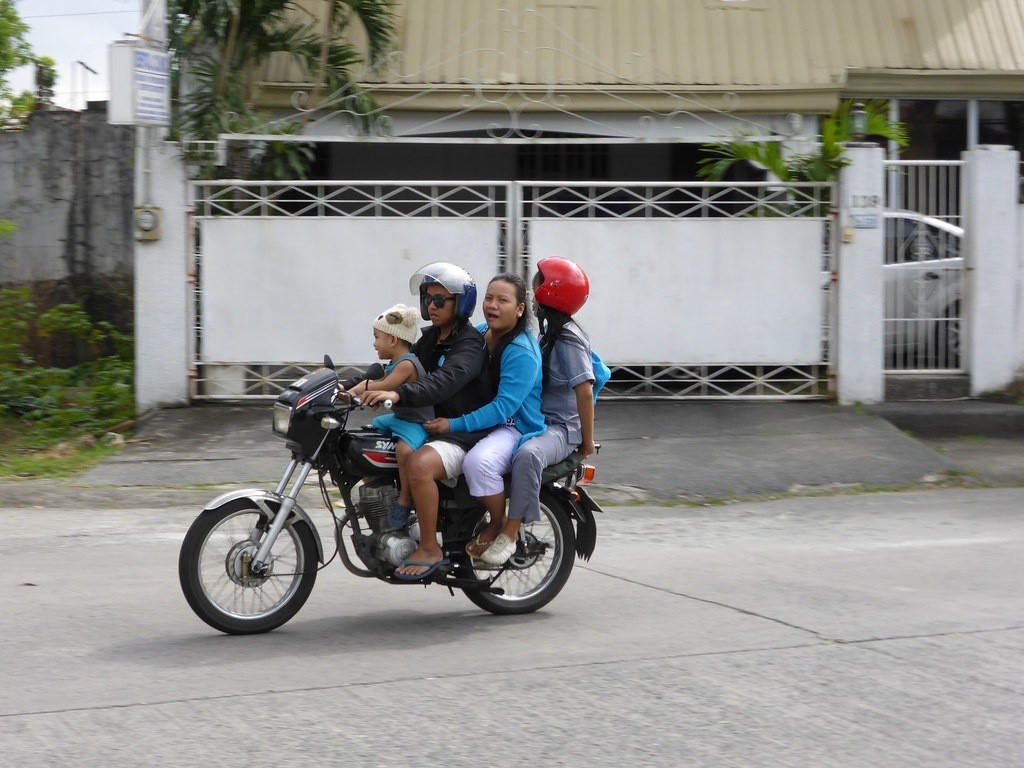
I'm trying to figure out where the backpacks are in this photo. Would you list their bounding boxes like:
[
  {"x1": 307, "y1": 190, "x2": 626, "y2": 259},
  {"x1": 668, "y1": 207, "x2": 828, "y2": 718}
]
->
[{"x1": 592, "y1": 350, "x2": 612, "y2": 406}]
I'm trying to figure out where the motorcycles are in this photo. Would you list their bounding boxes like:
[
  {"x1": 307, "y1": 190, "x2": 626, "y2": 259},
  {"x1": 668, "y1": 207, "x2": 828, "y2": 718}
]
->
[{"x1": 178, "y1": 355, "x2": 605, "y2": 635}]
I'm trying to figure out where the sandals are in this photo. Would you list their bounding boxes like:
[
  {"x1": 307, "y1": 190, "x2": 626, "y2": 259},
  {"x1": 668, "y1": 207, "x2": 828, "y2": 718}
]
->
[{"x1": 387, "y1": 500, "x2": 412, "y2": 526}]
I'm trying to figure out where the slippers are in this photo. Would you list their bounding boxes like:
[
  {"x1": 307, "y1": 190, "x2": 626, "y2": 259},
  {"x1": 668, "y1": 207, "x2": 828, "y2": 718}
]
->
[
  {"x1": 394, "y1": 557, "x2": 450, "y2": 580},
  {"x1": 465, "y1": 532, "x2": 495, "y2": 560}
]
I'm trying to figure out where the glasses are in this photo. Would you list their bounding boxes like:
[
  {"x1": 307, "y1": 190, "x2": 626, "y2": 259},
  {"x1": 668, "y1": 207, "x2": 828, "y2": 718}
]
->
[{"x1": 424, "y1": 293, "x2": 455, "y2": 310}]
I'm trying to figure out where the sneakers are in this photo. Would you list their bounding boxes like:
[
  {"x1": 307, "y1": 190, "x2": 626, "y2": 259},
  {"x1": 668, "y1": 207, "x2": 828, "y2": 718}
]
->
[{"x1": 480, "y1": 534, "x2": 517, "y2": 565}]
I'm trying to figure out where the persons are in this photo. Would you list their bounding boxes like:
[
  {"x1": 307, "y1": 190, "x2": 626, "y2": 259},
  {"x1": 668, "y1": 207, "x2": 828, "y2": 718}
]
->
[
  {"x1": 338, "y1": 261, "x2": 499, "y2": 580},
  {"x1": 422, "y1": 272, "x2": 547, "y2": 558},
  {"x1": 347, "y1": 303, "x2": 435, "y2": 525},
  {"x1": 481, "y1": 256, "x2": 595, "y2": 564}
]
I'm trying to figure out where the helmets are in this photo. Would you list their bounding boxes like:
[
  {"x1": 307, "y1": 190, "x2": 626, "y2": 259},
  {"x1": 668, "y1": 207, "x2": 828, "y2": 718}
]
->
[
  {"x1": 418, "y1": 268, "x2": 477, "y2": 318},
  {"x1": 535, "y1": 255, "x2": 589, "y2": 316}
]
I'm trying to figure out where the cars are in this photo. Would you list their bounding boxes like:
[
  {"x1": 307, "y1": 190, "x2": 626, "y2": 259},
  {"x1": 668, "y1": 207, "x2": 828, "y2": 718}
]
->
[{"x1": 821, "y1": 209, "x2": 966, "y2": 367}]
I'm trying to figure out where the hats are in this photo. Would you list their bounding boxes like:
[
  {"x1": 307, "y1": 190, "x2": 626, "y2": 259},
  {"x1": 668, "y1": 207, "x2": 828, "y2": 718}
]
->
[{"x1": 372, "y1": 302, "x2": 421, "y2": 345}]
